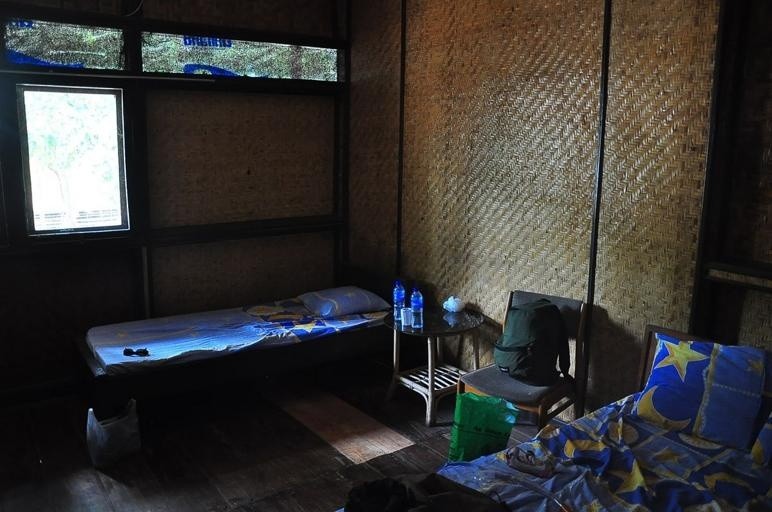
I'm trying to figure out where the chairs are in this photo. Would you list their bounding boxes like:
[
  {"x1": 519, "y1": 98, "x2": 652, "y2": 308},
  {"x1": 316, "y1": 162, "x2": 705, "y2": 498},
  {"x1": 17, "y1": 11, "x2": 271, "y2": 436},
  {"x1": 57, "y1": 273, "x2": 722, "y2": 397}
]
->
[{"x1": 451, "y1": 290, "x2": 584, "y2": 446}]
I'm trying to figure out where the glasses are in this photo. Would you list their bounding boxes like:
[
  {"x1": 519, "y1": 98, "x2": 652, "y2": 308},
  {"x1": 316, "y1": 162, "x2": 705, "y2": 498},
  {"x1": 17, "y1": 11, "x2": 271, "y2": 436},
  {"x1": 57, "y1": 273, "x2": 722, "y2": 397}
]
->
[{"x1": 124, "y1": 347, "x2": 149, "y2": 356}]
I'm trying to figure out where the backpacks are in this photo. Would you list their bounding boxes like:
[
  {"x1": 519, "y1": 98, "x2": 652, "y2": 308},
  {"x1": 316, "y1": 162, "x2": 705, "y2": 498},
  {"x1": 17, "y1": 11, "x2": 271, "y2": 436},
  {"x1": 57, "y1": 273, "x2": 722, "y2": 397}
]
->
[{"x1": 494, "y1": 298, "x2": 576, "y2": 387}]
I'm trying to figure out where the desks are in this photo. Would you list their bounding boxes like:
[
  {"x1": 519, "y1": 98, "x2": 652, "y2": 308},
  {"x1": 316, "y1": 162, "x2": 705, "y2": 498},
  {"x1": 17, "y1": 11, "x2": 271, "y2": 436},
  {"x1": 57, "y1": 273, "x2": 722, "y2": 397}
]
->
[{"x1": 382, "y1": 309, "x2": 485, "y2": 425}]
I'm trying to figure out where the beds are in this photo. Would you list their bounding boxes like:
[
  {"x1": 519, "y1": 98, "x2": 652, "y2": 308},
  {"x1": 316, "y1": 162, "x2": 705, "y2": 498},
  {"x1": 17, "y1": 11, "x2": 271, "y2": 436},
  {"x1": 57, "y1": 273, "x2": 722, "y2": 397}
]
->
[
  {"x1": 86, "y1": 287, "x2": 393, "y2": 460},
  {"x1": 331, "y1": 324, "x2": 772, "y2": 510}
]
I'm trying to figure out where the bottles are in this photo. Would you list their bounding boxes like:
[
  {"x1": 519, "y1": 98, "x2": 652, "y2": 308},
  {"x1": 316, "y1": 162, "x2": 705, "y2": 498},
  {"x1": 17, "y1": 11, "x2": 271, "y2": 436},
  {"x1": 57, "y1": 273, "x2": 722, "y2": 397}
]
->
[
  {"x1": 410, "y1": 288, "x2": 424, "y2": 329},
  {"x1": 393, "y1": 281, "x2": 405, "y2": 324}
]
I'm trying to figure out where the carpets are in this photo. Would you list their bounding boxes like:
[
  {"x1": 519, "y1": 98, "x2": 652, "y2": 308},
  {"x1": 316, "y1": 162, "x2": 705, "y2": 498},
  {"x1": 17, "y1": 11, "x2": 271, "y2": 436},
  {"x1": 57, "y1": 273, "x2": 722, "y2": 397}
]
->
[{"x1": 281, "y1": 396, "x2": 416, "y2": 465}]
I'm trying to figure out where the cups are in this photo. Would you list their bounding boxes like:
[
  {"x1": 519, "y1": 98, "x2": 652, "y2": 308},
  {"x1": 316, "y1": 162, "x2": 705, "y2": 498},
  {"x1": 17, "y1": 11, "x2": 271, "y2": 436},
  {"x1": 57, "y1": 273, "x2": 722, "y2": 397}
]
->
[{"x1": 400, "y1": 308, "x2": 413, "y2": 326}]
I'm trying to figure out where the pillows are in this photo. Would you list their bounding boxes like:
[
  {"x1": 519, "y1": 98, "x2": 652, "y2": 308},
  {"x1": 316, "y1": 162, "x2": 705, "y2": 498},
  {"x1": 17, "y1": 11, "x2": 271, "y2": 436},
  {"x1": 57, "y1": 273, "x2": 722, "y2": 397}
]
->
[
  {"x1": 299, "y1": 289, "x2": 389, "y2": 317},
  {"x1": 629, "y1": 333, "x2": 765, "y2": 453}
]
